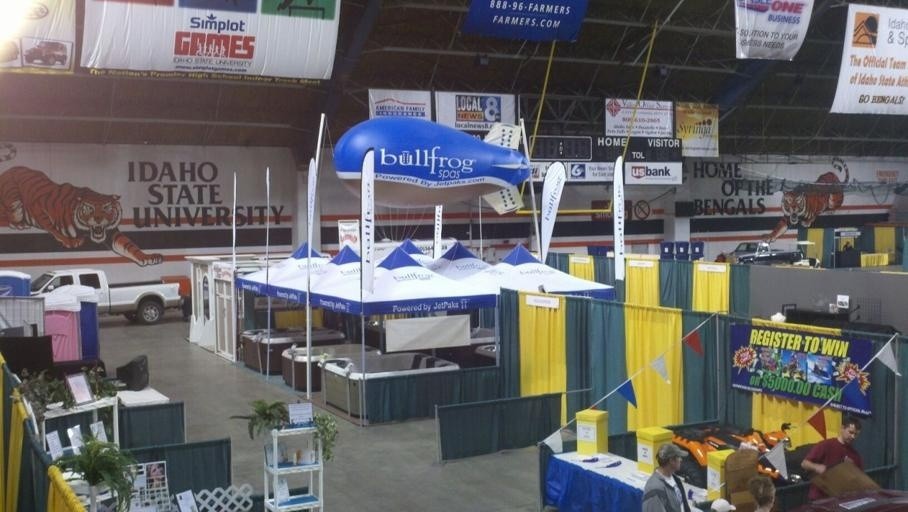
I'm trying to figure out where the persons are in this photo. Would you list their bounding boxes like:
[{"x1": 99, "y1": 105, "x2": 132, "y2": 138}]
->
[
  {"x1": 800, "y1": 418, "x2": 862, "y2": 503},
  {"x1": 642, "y1": 444, "x2": 690, "y2": 512},
  {"x1": 749, "y1": 476, "x2": 776, "y2": 512}
]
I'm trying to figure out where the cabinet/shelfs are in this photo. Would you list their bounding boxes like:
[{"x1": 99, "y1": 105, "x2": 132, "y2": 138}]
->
[{"x1": 262, "y1": 423, "x2": 324, "y2": 512}]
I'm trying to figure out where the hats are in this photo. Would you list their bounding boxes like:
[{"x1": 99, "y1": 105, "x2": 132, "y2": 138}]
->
[
  {"x1": 711, "y1": 499, "x2": 737, "y2": 512},
  {"x1": 656, "y1": 444, "x2": 689, "y2": 462}
]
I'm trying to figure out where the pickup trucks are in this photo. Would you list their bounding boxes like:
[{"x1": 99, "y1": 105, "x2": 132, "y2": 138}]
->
[
  {"x1": 30, "y1": 268, "x2": 184, "y2": 325},
  {"x1": 728, "y1": 242, "x2": 804, "y2": 265}
]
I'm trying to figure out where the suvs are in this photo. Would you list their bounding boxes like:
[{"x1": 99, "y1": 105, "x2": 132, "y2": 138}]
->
[{"x1": 24, "y1": 41, "x2": 68, "y2": 65}]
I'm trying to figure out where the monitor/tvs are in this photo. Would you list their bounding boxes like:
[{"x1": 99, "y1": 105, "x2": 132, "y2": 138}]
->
[{"x1": 116, "y1": 355, "x2": 148, "y2": 392}]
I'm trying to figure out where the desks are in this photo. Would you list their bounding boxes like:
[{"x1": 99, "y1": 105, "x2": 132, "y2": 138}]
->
[{"x1": 551, "y1": 449, "x2": 708, "y2": 512}]
